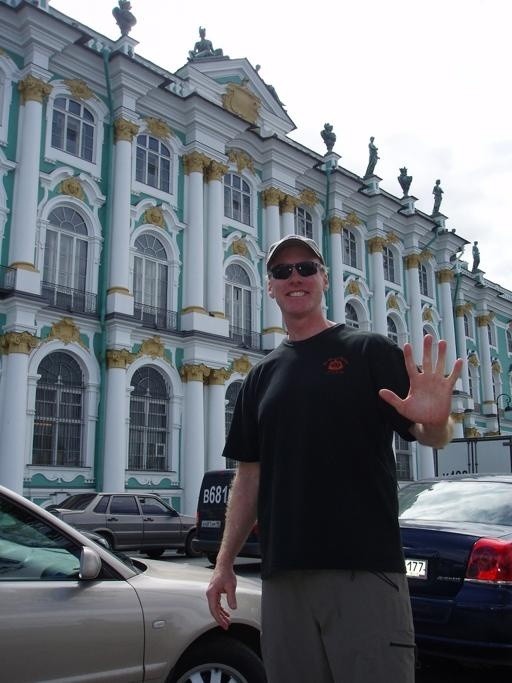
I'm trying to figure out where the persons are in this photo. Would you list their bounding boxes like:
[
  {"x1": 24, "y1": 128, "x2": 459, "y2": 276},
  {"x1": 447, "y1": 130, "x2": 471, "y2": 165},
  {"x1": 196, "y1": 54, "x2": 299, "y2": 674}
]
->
[
  {"x1": 470, "y1": 240, "x2": 483, "y2": 272},
  {"x1": 362, "y1": 134, "x2": 382, "y2": 178},
  {"x1": 430, "y1": 177, "x2": 444, "y2": 215},
  {"x1": 186, "y1": 21, "x2": 222, "y2": 57},
  {"x1": 204, "y1": 231, "x2": 465, "y2": 683}
]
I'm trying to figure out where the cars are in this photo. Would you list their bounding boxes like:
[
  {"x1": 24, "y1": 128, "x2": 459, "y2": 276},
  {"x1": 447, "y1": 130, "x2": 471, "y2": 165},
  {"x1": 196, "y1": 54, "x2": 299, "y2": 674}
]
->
[
  {"x1": 46, "y1": 491, "x2": 206, "y2": 558},
  {"x1": 397, "y1": 472, "x2": 511, "y2": 681},
  {"x1": 0, "y1": 485, "x2": 264, "y2": 682}
]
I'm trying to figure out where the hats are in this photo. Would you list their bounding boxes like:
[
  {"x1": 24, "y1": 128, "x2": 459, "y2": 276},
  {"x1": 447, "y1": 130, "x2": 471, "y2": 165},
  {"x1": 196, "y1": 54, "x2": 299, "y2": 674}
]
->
[{"x1": 266, "y1": 235, "x2": 325, "y2": 270}]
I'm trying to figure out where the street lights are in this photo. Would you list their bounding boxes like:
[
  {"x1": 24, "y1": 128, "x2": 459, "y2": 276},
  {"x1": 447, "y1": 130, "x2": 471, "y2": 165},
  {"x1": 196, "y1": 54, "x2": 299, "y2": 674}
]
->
[{"x1": 496, "y1": 393, "x2": 512, "y2": 435}]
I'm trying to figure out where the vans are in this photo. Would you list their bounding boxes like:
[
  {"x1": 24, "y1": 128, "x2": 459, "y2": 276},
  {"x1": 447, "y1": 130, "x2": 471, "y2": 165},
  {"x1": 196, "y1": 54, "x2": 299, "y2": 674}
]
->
[{"x1": 191, "y1": 467, "x2": 262, "y2": 564}]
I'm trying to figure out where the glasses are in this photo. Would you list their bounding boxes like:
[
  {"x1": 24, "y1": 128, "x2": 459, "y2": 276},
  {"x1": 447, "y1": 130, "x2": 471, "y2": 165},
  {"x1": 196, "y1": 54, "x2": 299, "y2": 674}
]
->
[{"x1": 271, "y1": 262, "x2": 318, "y2": 279}]
